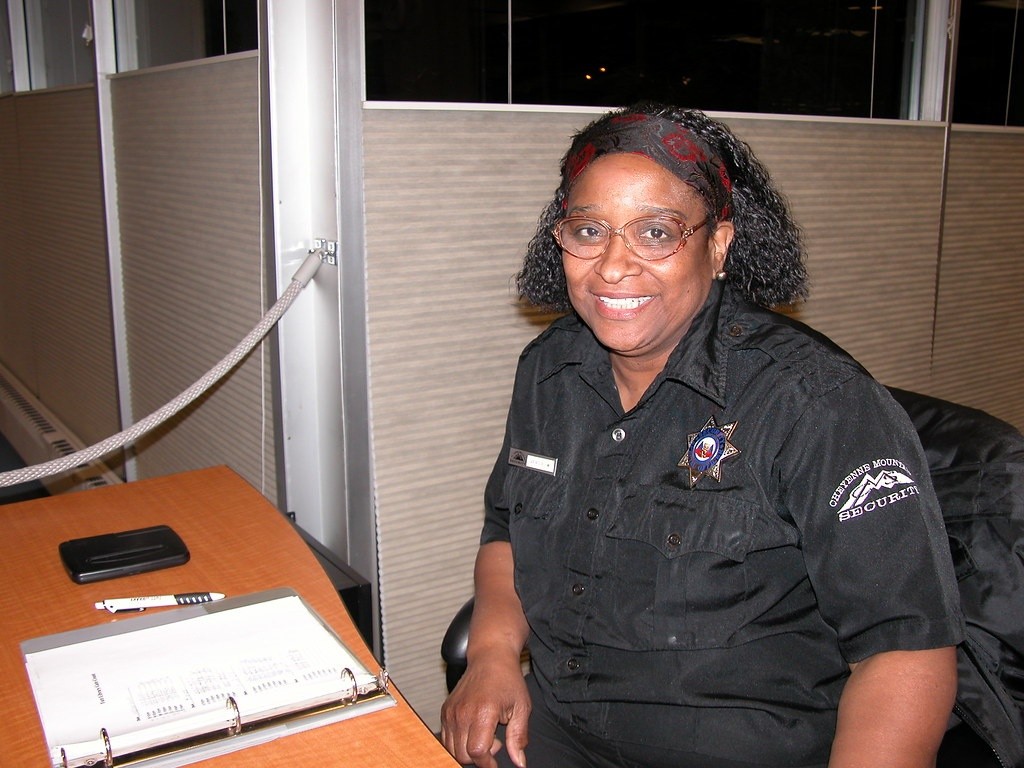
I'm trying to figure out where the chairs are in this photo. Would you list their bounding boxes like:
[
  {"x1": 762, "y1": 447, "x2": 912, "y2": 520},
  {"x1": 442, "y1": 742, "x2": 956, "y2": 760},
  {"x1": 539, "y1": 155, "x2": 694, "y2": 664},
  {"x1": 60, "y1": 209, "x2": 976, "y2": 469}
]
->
[{"x1": 440, "y1": 385, "x2": 1024, "y2": 768}]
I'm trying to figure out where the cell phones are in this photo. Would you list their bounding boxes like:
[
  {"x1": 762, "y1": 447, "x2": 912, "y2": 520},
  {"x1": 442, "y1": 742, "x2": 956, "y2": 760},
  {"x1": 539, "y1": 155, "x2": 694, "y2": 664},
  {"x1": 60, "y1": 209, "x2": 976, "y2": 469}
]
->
[{"x1": 59, "y1": 525, "x2": 191, "y2": 585}]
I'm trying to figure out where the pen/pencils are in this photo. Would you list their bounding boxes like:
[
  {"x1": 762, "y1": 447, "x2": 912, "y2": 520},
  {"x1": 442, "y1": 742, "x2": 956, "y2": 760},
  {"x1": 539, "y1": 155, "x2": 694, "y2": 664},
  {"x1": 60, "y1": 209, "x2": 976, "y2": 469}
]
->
[{"x1": 95, "y1": 592, "x2": 225, "y2": 613}]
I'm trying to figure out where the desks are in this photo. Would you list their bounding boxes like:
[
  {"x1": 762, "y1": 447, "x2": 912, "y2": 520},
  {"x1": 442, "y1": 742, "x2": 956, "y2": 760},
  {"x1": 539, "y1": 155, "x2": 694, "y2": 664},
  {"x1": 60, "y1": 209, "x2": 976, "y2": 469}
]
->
[{"x1": 0, "y1": 464, "x2": 466, "y2": 768}]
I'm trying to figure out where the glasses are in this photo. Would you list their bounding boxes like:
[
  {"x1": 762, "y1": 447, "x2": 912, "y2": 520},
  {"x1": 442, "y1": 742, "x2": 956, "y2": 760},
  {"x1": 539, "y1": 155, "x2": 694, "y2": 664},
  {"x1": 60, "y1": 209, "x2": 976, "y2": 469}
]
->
[{"x1": 550, "y1": 215, "x2": 711, "y2": 260}]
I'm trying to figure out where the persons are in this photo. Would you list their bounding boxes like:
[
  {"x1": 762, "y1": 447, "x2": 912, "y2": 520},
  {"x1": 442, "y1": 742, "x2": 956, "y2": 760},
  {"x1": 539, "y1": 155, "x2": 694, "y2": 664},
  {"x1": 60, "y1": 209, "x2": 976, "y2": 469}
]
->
[{"x1": 439, "y1": 103, "x2": 971, "y2": 768}]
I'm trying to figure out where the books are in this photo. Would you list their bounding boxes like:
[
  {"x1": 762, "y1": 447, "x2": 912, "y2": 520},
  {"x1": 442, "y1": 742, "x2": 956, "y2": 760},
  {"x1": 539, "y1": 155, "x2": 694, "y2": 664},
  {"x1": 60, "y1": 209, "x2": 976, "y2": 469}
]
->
[{"x1": 21, "y1": 587, "x2": 398, "y2": 768}]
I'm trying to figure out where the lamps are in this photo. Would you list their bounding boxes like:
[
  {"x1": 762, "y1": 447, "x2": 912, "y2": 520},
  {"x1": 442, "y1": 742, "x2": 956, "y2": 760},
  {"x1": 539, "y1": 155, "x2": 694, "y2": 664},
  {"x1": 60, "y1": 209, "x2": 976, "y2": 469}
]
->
[{"x1": 81, "y1": 26, "x2": 94, "y2": 47}]
